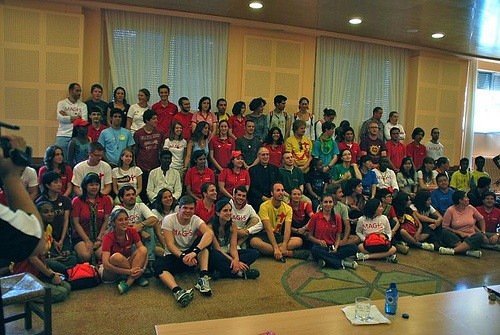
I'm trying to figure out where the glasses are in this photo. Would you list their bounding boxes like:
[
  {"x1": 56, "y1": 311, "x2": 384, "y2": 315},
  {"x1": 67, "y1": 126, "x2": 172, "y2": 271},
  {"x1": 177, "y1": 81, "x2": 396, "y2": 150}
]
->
[{"x1": 391, "y1": 132, "x2": 400, "y2": 135}]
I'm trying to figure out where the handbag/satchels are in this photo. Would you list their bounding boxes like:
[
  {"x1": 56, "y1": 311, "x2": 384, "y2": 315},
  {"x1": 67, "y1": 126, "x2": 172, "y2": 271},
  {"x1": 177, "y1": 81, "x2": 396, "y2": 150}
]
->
[{"x1": 64, "y1": 262, "x2": 101, "y2": 291}]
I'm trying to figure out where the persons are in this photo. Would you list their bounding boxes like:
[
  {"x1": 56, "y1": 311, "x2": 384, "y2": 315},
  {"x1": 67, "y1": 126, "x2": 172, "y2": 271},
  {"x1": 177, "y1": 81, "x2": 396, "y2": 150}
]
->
[
  {"x1": 154, "y1": 197, "x2": 215, "y2": 308},
  {"x1": 0, "y1": 82, "x2": 500, "y2": 262},
  {"x1": 203, "y1": 201, "x2": 261, "y2": 280},
  {"x1": 98, "y1": 209, "x2": 151, "y2": 294},
  {"x1": 0, "y1": 134, "x2": 43, "y2": 335},
  {"x1": 13, "y1": 202, "x2": 71, "y2": 303},
  {"x1": 306, "y1": 195, "x2": 359, "y2": 269}
]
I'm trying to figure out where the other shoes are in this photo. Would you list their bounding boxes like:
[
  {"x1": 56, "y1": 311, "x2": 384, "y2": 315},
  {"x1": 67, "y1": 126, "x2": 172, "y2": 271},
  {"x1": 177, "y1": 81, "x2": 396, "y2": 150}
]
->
[
  {"x1": 136, "y1": 277, "x2": 148, "y2": 286},
  {"x1": 241, "y1": 268, "x2": 260, "y2": 279},
  {"x1": 294, "y1": 249, "x2": 311, "y2": 258},
  {"x1": 117, "y1": 280, "x2": 128, "y2": 294},
  {"x1": 213, "y1": 269, "x2": 223, "y2": 279}
]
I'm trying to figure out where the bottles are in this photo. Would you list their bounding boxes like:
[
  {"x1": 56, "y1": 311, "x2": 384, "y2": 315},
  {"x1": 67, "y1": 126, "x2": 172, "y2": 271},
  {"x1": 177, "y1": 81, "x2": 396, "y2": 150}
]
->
[{"x1": 384, "y1": 282, "x2": 398, "y2": 314}]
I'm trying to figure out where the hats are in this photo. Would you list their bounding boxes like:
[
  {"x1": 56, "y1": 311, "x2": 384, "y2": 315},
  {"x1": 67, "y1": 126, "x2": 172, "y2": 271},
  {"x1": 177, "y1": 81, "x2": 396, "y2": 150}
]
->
[
  {"x1": 230, "y1": 150, "x2": 246, "y2": 160},
  {"x1": 73, "y1": 118, "x2": 91, "y2": 127}
]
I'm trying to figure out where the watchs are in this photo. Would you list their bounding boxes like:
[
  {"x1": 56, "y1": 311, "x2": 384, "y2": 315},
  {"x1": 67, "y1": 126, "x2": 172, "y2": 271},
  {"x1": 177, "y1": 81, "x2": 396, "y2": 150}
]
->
[
  {"x1": 496, "y1": 233, "x2": 500, "y2": 236},
  {"x1": 141, "y1": 221, "x2": 146, "y2": 227},
  {"x1": 49, "y1": 272, "x2": 56, "y2": 279}
]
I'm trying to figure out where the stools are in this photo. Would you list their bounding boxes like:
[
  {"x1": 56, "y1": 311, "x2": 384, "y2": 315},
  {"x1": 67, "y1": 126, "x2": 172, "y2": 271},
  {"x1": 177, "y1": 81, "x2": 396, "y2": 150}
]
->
[{"x1": 0, "y1": 271, "x2": 51, "y2": 335}]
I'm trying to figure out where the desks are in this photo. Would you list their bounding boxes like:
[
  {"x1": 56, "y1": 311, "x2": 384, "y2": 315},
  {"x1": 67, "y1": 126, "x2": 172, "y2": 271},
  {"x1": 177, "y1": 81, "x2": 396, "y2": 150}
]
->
[{"x1": 155, "y1": 286, "x2": 500, "y2": 335}]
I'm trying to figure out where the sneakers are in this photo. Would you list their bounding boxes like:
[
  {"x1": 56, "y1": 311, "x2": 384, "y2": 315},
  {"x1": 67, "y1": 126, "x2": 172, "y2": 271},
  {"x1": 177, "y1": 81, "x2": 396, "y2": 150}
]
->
[
  {"x1": 341, "y1": 259, "x2": 358, "y2": 270},
  {"x1": 355, "y1": 253, "x2": 366, "y2": 261},
  {"x1": 317, "y1": 259, "x2": 326, "y2": 267},
  {"x1": 439, "y1": 247, "x2": 455, "y2": 255},
  {"x1": 421, "y1": 242, "x2": 434, "y2": 251},
  {"x1": 396, "y1": 244, "x2": 409, "y2": 254},
  {"x1": 194, "y1": 275, "x2": 212, "y2": 295},
  {"x1": 173, "y1": 287, "x2": 194, "y2": 307},
  {"x1": 466, "y1": 251, "x2": 482, "y2": 258},
  {"x1": 400, "y1": 241, "x2": 407, "y2": 246},
  {"x1": 385, "y1": 254, "x2": 397, "y2": 263}
]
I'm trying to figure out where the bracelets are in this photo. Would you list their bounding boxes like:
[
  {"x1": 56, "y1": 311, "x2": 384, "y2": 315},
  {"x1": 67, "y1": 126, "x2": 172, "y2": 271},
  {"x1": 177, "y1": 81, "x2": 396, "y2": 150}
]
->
[
  {"x1": 130, "y1": 269, "x2": 132, "y2": 276},
  {"x1": 327, "y1": 165, "x2": 332, "y2": 170},
  {"x1": 96, "y1": 237, "x2": 101, "y2": 240},
  {"x1": 78, "y1": 115, "x2": 81, "y2": 119},
  {"x1": 302, "y1": 226, "x2": 306, "y2": 229},
  {"x1": 481, "y1": 232, "x2": 486, "y2": 234},
  {"x1": 179, "y1": 252, "x2": 187, "y2": 260},
  {"x1": 296, "y1": 228, "x2": 298, "y2": 233},
  {"x1": 418, "y1": 228, "x2": 422, "y2": 230},
  {"x1": 192, "y1": 247, "x2": 201, "y2": 254}
]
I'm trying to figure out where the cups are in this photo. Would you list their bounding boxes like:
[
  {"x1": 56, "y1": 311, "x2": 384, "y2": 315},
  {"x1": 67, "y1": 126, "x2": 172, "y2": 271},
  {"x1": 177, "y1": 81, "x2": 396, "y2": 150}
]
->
[{"x1": 354, "y1": 297, "x2": 372, "y2": 319}]
